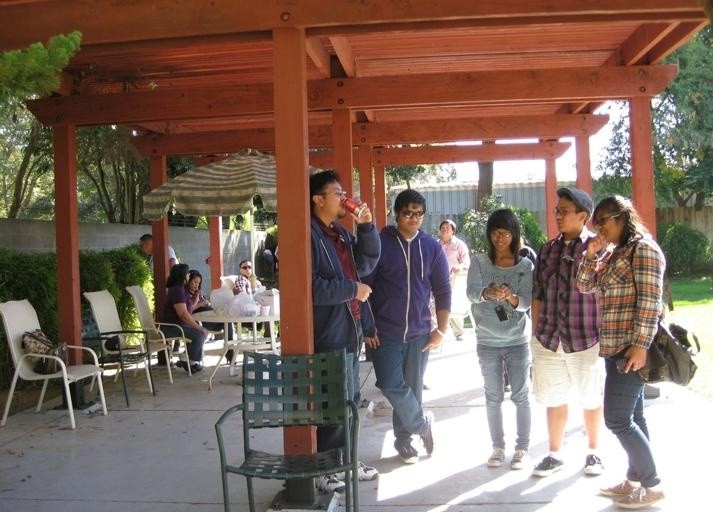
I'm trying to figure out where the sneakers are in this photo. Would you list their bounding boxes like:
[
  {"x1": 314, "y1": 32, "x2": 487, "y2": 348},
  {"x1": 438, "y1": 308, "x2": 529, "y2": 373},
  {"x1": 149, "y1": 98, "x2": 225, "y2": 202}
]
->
[
  {"x1": 314, "y1": 472, "x2": 346, "y2": 493},
  {"x1": 455, "y1": 333, "x2": 464, "y2": 341},
  {"x1": 598, "y1": 478, "x2": 640, "y2": 496},
  {"x1": 531, "y1": 454, "x2": 569, "y2": 477},
  {"x1": 613, "y1": 486, "x2": 667, "y2": 509},
  {"x1": 420, "y1": 410, "x2": 436, "y2": 456},
  {"x1": 334, "y1": 457, "x2": 379, "y2": 483},
  {"x1": 176, "y1": 360, "x2": 204, "y2": 375},
  {"x1": 509, "y1": 448, "x2": 529, "y2": 470},
  {"x1": 392, "y1": 442, "x2": 419, "y2": 464},
  {"x1": 486, "y1": 446, "x2": 506, "y2": 468},
  {"x1": 582, "y1": 454, "x2": 605, "y2": 475}
]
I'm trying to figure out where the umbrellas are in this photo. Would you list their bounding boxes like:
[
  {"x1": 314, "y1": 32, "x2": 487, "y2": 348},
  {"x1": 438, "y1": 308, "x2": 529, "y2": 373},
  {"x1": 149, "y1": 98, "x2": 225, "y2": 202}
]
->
[{"x1": 142, "y1": 146, "x2": 324, "y2": 354}]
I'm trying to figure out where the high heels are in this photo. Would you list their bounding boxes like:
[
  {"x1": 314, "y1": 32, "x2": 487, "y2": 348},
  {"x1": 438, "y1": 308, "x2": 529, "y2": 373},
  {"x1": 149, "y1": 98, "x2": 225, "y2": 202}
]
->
[{"x1": 224, "y1": 348, "x2": 237, "y2": 365}]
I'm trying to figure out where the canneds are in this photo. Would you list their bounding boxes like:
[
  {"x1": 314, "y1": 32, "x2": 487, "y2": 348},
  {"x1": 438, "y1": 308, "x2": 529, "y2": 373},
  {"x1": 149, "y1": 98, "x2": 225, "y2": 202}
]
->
[{"x1": 342, "y1": 198, "x2": 364, "y2": 218}]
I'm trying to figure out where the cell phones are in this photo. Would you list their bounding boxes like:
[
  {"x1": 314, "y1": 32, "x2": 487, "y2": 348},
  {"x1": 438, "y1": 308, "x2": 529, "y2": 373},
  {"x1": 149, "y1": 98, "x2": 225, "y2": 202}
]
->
[
  {"x1": 614, "y1": 358, "x2": 634, "y2": 374},
  {"x1": 495, "y1": 304, "x2": 508, "y2": 321}
]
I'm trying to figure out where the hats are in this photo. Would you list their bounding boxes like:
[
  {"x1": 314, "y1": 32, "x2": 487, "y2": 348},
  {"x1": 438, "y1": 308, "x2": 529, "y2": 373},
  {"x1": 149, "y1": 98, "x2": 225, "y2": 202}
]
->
[{"x1": 550, "y1": 184, "x2": 595, "y2": 226}]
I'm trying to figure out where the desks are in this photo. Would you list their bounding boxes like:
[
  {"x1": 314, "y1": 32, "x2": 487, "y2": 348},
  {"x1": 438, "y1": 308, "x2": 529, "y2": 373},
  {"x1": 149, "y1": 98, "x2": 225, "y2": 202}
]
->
[{"x1": 192, "y1": 309, "x2": 281, "y2": 390}]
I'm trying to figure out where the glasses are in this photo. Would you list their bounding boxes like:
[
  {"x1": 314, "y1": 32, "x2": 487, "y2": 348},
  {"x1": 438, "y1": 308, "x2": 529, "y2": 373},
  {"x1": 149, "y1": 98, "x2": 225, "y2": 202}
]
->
[
  {"x1": 314, "y1": 189, "x2": 347, "y2": 198},
  {"x1": 239, "y1": 265, "x2": 252, "y2": 270},
  {"x1": 397, "y1": 208, "x2": 425, "y2": 219},
  {"x1": 552, "y1": 205, "x2": 579, "y2": 214},
  {"x1": 594, "y1": 210, "x2": 624, "y2": 227},
  {"x1": 491, "y1": 228, "x2": 512, "y2": 238}
]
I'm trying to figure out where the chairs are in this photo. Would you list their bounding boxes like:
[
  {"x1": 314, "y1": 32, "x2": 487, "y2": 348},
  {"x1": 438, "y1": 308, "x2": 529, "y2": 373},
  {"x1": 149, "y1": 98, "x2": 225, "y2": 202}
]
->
[
  {"x1": 84, "y1": 290, "x2": 174, "y2": 394},
  {"x1": 1, "y1": 299, "x2": 108, "y2": 429},
  {"x1": 74, "y1": 331, "x2": 155, "y2": 407},
  {"x1": 214, "y1": 346, "x2": 361, "y2": 511},
  {"x1": 126, "y1": 285, "x2": 192, "y2": 384}
]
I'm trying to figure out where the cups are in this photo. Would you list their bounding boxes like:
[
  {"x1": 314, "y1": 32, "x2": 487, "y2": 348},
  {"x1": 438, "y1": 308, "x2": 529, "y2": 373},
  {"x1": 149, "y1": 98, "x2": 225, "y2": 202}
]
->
[{"x1": 261, "y1": 305, "x2": 271, "y2": 316}]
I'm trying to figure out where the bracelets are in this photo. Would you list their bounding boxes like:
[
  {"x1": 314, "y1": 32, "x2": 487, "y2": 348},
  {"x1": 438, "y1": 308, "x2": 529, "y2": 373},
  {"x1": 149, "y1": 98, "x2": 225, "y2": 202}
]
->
[
  {"x1": 483, "y1": 287, "x2": 492, "y2": 300},
  {"x1": 435, "y1": 327, "x2": 446, "y2": 338}
]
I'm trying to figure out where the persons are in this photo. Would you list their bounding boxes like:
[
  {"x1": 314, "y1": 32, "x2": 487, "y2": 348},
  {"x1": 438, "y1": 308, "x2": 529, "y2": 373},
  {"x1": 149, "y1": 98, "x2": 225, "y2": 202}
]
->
[
  {"x1": 436, "y1": 219, "x2": 470, "y2": 341},
  {"x1": 360, "y1": 188, "x2": 451, "y2": 465},
  {"x1": 504, "y1": 237, "x2": 537, "y2": 393},
  {"x1": 309, "y1": 168, "x2": 379, "y2": 495},
  {"x1": 574, "y1": 193, "x2": 665, "y2": 509},
  {"x1": 528, "y1": 186, "x2": 608, "y2": 479},
  {"x1": 465, "y1": 208, "x2": 536, "y2": 470},
  {"x1": 138, "y1": 233, "x2": 261, "y2": 375}
]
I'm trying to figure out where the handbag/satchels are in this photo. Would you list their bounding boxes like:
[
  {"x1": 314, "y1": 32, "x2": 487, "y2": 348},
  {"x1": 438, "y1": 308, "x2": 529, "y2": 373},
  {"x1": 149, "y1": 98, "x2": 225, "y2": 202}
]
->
[
  {"x1": 21, "y1": 329, "x2": 55, "y2": 366},
  {"x1": 33, "y1": 341, "x2": 71, "y2": 376},
  {"x1": 644, "y1": 317, "x2": 702, "y2": 386}
]
